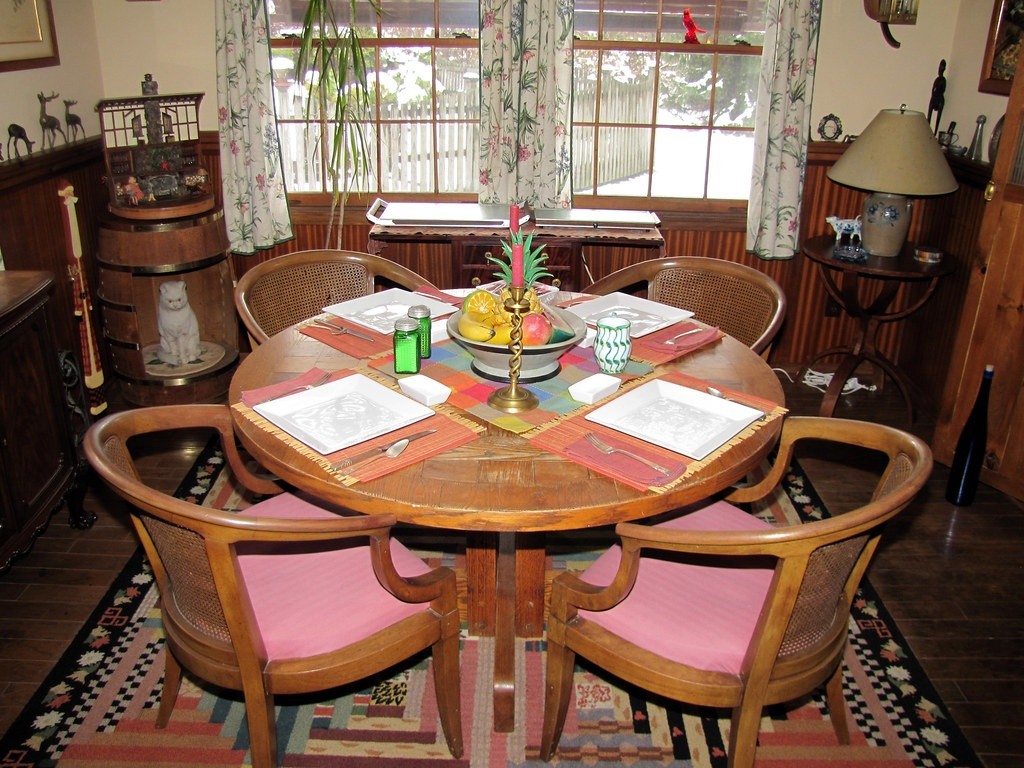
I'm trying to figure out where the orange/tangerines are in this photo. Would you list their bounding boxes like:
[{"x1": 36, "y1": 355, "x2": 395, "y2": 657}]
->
[{"x1": 463, "y1": 289, "x2": 496, "y2": 316}]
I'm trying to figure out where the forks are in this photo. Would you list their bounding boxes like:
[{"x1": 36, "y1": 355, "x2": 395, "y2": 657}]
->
[
  {"x1": 584, "y1": 432, "x2": 672, "y2": 476},
  {"x1": 254, "y1": 372, "x2": 332, "y2": 403}
]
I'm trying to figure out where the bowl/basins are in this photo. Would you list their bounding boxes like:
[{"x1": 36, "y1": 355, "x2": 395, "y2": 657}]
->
[{"x1": 446, "y1": 304, "x2": 588, "y2": 383}]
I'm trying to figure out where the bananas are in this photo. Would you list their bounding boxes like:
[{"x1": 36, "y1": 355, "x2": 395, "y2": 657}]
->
[{"x1": 459, "y1": 310, "x2": 519, "y2": 345}]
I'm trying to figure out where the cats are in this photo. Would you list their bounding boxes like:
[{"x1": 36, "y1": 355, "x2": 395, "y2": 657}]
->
[{"x1": 155, "y1": 281, "x2": 202, "y2": 366}]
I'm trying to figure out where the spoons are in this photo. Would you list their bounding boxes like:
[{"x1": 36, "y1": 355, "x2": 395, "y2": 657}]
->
[
  {"x1": 306, "y1": 324, "x2": 347, "y2": 335},
  {"x1": 706, "y1": 386, "x2": 771, "y2": 416},
  {"x1": 341, "y1": 439, "x2": 409, "y2": 474}
]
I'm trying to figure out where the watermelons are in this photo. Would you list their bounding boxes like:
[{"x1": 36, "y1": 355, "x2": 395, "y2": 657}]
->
[{"x1": 540, "y1": 303, "x2": 575, "y2": 343}]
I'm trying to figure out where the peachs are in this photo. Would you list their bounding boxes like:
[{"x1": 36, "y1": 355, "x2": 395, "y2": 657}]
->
[{"x1": 519, "y1": 313, "x2": 552, "y2": 347}]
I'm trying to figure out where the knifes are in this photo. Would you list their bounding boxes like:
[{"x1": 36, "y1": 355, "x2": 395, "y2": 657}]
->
[
  {"x1": 329, "y1": 428, "x2": 439, "y2": 471},
  {"x1": 314, "y1": 318, "x2": 375, "y2": 342}
]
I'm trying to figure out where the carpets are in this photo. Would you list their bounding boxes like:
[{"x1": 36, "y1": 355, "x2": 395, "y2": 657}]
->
[{"x1": 0, "y1": 434, "x2": 985, "y2": 768}]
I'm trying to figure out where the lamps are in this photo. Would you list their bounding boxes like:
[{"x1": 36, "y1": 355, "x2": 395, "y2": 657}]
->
[{"x1": 826, "y1": 103, "x2": 958, "y2": 257}]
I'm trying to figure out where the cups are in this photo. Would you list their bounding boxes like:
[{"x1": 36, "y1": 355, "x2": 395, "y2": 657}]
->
[
  {"x1": 949, "y1": 145, "x2": 967, "y2": 156},
  {"x1": 938, "y1": 130, "x2": 959, "y2": 145},
  {"x1": 593, "y1": 312, "x2": 633, "y2": 375}
]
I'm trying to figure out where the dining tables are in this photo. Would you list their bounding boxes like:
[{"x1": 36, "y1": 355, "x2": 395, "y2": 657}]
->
[{"x1": 228, "y1": 289, "x2": 786, "y2": 734}]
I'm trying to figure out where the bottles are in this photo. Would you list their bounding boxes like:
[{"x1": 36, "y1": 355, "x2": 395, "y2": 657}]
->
[
  {"x1": 945, "y1": 370, "x2": 994, "y2": 506},
  {"x1": 407, "y1": 303, "x2": 432, "y2": 359},
  {"x1": 393, "y1": 318, "x2": 421, "y2": 374},
  {"x1": 966, "y1": 115, "x2": 986, "y2": 161}
]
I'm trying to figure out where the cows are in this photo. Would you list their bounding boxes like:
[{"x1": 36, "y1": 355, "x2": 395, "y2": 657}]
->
[{"x1": 826, "y1": 215, "x2": 862, "y2": 242}]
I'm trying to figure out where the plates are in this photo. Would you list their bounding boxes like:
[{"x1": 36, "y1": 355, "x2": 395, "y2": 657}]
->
[
  {"x1": 562, "y1": 292, "x2": 695, "y2": 338},
  {"x1": 251, "y1": 373, "x2": 436, "y2": 457},
  {"x1": 321, "y1": 288, "x2": 459, "y2": 335},
  {"x1": 583, "y1": 377, "x2": 765, "y2": 461},
  {"x1": 475, "y1": 280, "x2": 559, "y2": 301}
]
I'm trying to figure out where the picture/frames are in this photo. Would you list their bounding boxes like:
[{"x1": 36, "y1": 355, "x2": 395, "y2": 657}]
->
[
  {"x1": 978, "y1": 0, "x2": 1024, "y2": 97},
  {"x1": 0, "y1": 0, "x2": 60, "y2": 74}
]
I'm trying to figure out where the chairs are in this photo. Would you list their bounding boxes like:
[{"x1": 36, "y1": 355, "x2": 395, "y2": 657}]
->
[
  {"x1": 235, "y1": 249, "x2": 440, "y2": 352},
  {"x1": 540, "y1": 416, "x2": 933, "y2": 768},
  {"x1": 84, "y1": 403, "x2": 464, "y2": 768},
  {"x1": 580, "y1": 257, "x2": 786, "y2": 364}
]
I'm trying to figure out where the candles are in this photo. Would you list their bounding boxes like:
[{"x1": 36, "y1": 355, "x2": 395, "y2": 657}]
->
[
  {"x1": 510, "y1": 202, "x2": 519, "y2": 234},
  {"x1": 512, "y1": 242, "x2": 524, "y2": 287}
]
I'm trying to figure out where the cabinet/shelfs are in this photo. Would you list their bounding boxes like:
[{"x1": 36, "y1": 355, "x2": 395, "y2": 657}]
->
[
  {"x1": 364, "y1": 197, "x2": 665, "y2": 299},
  {"x1": 0, "y1": 270, "x2": 98, "y2": 574}
]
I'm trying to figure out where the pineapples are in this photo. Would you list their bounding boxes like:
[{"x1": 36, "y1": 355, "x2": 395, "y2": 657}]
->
[{"x1": 488, "y1": 227, "x2": 554, "y2": 322}]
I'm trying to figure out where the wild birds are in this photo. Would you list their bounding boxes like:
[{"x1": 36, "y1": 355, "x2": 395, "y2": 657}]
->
[{"x1": 682, "y1": 9, "x2": 708, "y2": 35}]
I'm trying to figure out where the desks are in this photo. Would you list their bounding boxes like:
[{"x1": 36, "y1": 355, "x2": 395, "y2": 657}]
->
[{"x1": 793, "y1": 233, "x2": 960, "y2": 426}]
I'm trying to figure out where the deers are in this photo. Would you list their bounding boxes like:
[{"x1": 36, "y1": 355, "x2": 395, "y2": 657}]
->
[{"x1": 7, "y1": 90, "x2": 87, "y2": 162}]
